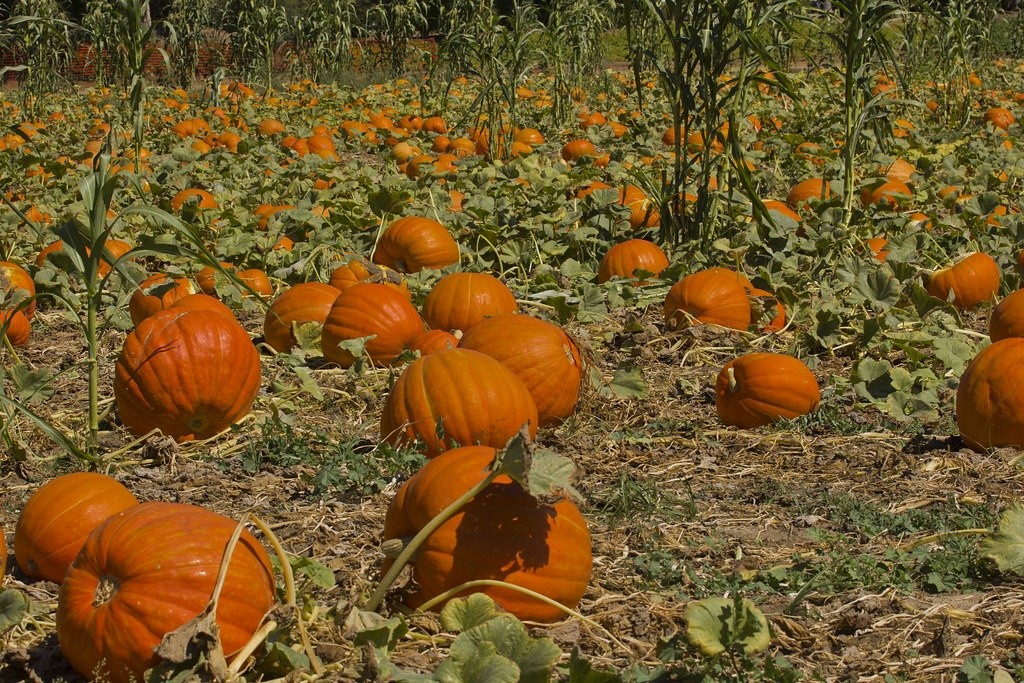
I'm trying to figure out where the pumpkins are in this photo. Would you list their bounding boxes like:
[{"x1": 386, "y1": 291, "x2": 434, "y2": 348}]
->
[{"x1": 0, "y1": 60, "x2": 1024, "y2": 683}]
